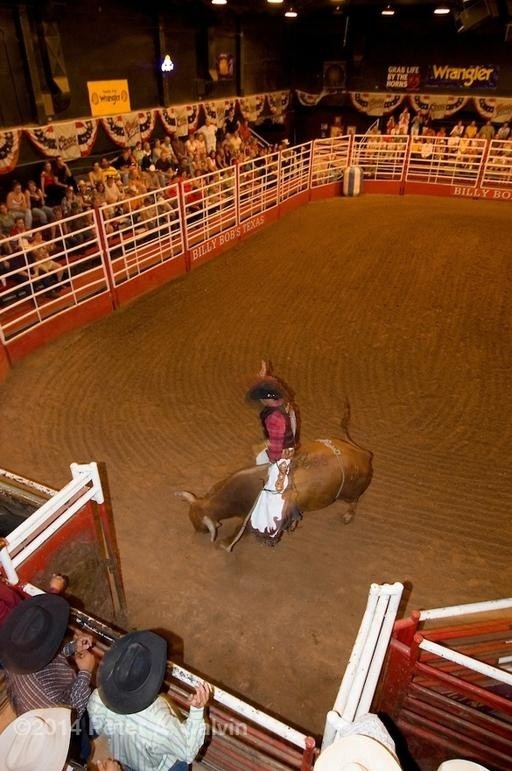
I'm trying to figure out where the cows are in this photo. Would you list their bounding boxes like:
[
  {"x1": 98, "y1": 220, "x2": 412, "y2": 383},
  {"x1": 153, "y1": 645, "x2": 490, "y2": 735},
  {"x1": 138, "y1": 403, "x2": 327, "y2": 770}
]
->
[{"x1": 174, "y1": 401, "x2": 374, "y2": 552}]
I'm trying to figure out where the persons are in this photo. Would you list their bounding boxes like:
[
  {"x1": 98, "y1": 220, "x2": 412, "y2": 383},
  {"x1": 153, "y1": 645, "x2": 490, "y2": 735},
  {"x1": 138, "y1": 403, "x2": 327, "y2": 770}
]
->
[
  {"x1": 0, "y1": 706, "x2": 121, "y2": 771},
  {"x1": 1, "y1": 536, "x2": 69, "y2": 629},
  {"x1": 312, "y1": 714, "x2": 401, "y2": 771},
  {"x1": 2, "y1": 595, "x2": 98, "y2": 765},
  {"x1": 88, "y1": 631, "x2": 212, "y2": 771},
  {"x1": 362, "y1": 106, "x2": 512, "y2": 181},
  {"x1": 244, "y1": 357, "x2": 300, "y2": 546},
  {"x1": 0, "y1": 108, "x2": 307, "y2": 300}
]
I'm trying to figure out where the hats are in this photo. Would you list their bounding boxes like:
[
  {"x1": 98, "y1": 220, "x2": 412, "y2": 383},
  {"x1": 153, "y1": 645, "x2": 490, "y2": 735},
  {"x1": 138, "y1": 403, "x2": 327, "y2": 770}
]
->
[
  {"x1": 96, "y1": 629, "x2": 167, "y2": 715},
  {"x1": 316, "y1": 735, "x2": 403, "y2": 771},
  {"x1": 1, "y1": 707, "x2": 72, "y2": 771},
  {"x1": 0, "y1": 594, "x2": 70, "y2": 674},
  {"x1": 250, "y1": 379, "x2": 285, "y2": 400}
]
